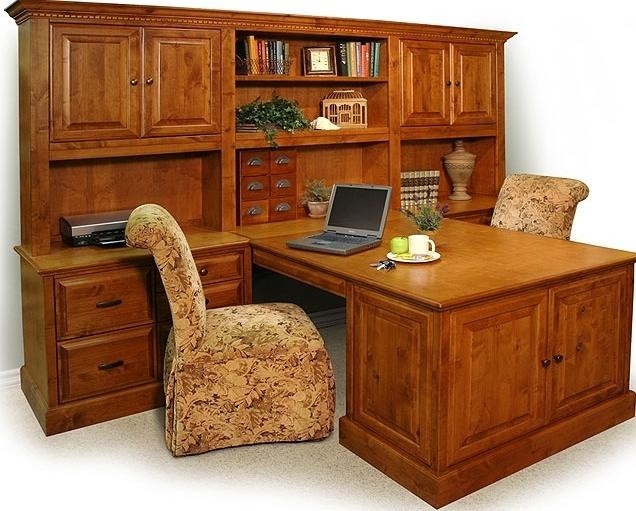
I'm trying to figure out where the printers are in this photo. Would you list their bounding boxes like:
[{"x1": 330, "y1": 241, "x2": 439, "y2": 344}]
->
[{"x1": 59, "y1": 210, "x2": 132, "y2": 246}]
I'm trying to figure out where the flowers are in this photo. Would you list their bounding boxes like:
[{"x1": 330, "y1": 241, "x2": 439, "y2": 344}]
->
[{"x1": 399, "y1": 191, "x2": 450, "y2": 231}]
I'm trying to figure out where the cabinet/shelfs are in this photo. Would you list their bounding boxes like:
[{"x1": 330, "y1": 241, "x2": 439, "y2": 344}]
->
[
  {"x1": 14, "y1": 225, "x2": 252, "y2": 438},
  {"x1": 393, "y1": 21, "x2": 517, "y2": 209},
  {"x1": 236, "y1": 147, "x2": 297, "y2": 225},
  {"x1": 229, "y1": 11, "x2": 395, "y2": 230},
  {"x1": 5, "y1": 0, "x2": 228, "y2": 258}
]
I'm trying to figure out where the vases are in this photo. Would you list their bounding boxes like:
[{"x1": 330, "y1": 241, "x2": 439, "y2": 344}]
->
[{"x1": 418, "y1": 229, "x2": 437, "y2": 250}]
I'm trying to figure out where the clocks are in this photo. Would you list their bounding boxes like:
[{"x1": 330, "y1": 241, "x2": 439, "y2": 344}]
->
[{"x1": 303, "y1": 46, "x2": 338, "y2": 77}]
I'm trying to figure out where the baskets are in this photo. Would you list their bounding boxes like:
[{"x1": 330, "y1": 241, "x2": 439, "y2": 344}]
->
[{"x1": 234, "y1": 58, "x2": 294, "y2": 75}]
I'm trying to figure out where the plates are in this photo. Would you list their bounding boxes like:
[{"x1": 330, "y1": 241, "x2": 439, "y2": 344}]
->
[{"x1": 387, "y1": 251, "x2": 442, "y2": 264}]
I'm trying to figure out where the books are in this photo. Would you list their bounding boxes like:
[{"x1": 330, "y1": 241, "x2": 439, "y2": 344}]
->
[
  {"x1": 399, "y1": 170, "x2": 440, "y2": 212},
  {"x1": 235, "y1": 34, "x2": 291, "y2": 75},
  {"x1": 327, "y1": 41, "x2": 381, "y2": 78}
]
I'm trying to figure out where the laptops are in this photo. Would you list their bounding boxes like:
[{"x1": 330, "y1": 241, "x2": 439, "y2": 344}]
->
[{"x1": 286, "y1": 183, "x2": 392, "y2": 255}]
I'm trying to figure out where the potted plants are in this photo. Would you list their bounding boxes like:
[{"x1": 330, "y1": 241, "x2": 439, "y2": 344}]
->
[{"x1": 301, "y1": 179, "x2": 333, "y2": 218}]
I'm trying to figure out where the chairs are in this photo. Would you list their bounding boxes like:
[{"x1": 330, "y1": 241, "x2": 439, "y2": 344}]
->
[
  {"x1": 124, "y1": 204, "x2": 336, "y2": 457},
  {"x1": 490, "y1": 174, "x2": 589, "y2": 240}
]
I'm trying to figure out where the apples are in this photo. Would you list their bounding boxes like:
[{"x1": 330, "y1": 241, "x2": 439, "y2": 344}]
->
[{"x1": 390, "y1": 236, "x2": 409, "y2": 254}]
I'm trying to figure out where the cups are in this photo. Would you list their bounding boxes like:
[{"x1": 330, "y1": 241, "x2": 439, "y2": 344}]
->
[{"x1": 408, "y1": 234, "x2": 436, "y2": 257}]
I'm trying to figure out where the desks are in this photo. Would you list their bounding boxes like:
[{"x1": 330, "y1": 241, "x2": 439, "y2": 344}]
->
[{"x1": 229, "y1": 213, "x2": 636, "y2": 511}]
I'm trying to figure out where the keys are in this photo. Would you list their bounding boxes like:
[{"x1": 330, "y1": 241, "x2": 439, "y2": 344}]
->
[{"x1": 368, "y1": 259, "x2": 399, "y2": 273}]
